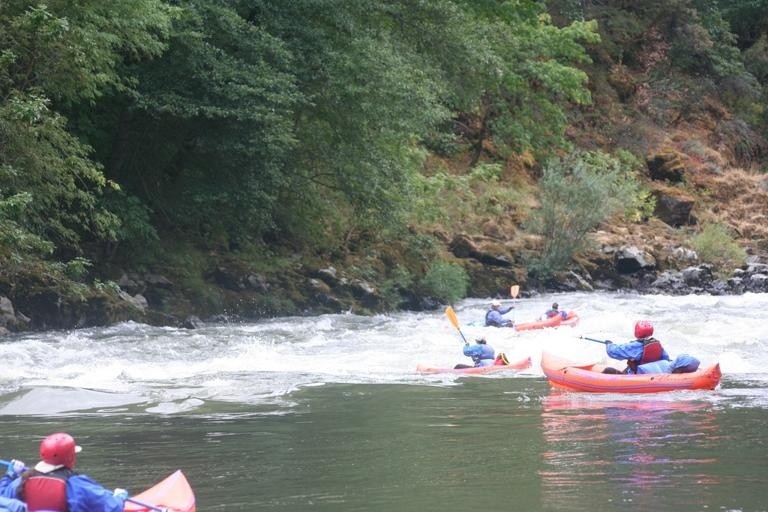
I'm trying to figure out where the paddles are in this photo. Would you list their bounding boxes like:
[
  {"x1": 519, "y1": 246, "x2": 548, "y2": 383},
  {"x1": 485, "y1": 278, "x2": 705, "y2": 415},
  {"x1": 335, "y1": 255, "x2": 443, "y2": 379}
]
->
[
  {"x1": 445, "y1": 305, "x2": 468, "y2": 343},
  {"x1": 511, "y1": 285, "x2": 520, "y2": 318}
]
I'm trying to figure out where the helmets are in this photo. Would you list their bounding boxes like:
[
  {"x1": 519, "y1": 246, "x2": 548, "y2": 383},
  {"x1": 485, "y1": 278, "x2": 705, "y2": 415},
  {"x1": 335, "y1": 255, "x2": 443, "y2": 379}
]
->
[
  {"x1": 635, "y1": 321, "x2": 653, "y2": 338},
  {"x1": 40, "y1": 433, "x2": 82, "y2": 466}
]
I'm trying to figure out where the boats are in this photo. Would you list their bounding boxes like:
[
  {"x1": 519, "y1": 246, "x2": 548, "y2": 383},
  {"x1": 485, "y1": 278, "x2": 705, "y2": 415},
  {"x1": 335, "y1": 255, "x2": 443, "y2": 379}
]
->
[
  {"x1": 416, "y1": 355, "x2": 531, "y2": 376},
  {"x1": 541, "y1": 352, "x2": 723, "y2": 394},
  {"x1": 119, "y1": 465, "x2": 197, "y2": 512},
  {"x1": 513, "y1": 310, "x2": 578, "y2": 334}
]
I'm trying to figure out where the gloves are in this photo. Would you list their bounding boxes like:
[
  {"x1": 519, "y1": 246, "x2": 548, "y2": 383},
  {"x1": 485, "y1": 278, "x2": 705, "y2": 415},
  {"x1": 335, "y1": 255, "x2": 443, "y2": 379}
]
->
[
  {"x1": 113, "y1": 488, "x2": 127, "y2": 500},
  {"x1": 6, "y1": 459, "x2": 24, "y2": 478}
]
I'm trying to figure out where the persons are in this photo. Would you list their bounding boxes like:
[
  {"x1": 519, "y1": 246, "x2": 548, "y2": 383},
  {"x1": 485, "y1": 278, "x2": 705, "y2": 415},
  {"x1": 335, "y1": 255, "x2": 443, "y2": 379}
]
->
[
  {"x1": 485, "y1": 298, "x2": 515, "y2": 328},
  {"x1": 540, "y1": 302, "x2": 567, "y2": 320},
  {"x1": 0, "y1": 432, "x2": 128, "y2": 512},
  {"x1": 454, "y1": 335, "x2": 496, "y2": 369},
  {"x1": 602, "y1": 320, "x2": 671, "y2": 374}
]
